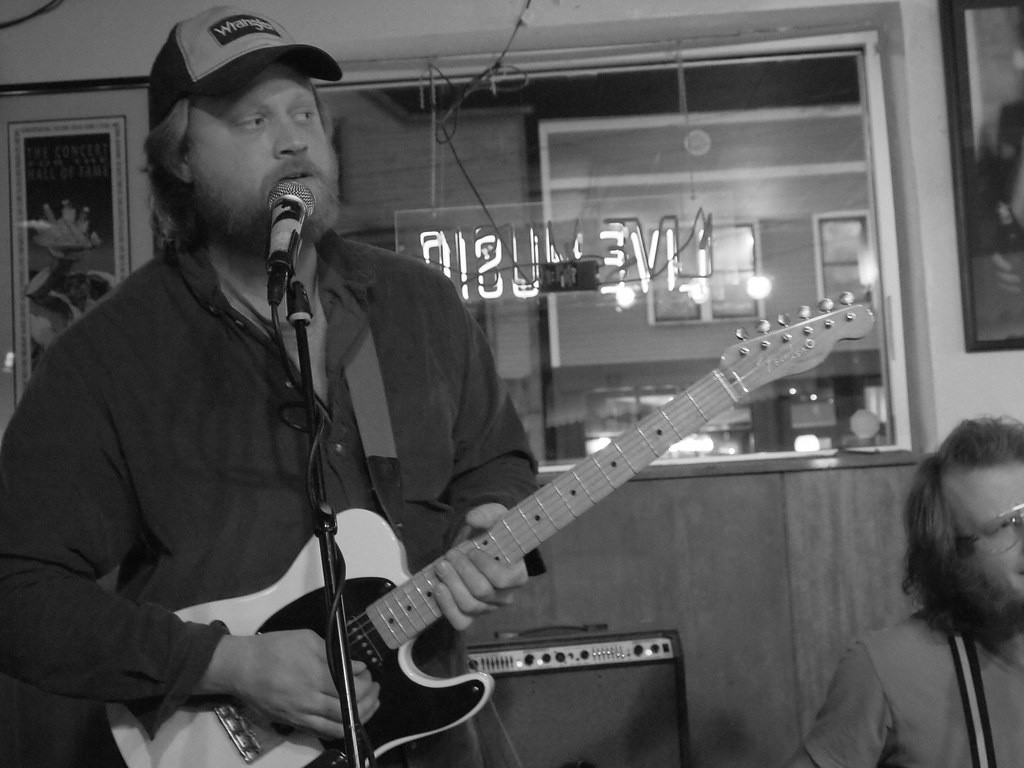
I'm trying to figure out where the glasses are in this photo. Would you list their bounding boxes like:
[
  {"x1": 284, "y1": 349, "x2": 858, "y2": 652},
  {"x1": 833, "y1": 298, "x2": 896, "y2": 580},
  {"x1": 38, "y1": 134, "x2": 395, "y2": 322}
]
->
[{"x1": 952, "y1": 503, "x2": 1024, "y2": 558}]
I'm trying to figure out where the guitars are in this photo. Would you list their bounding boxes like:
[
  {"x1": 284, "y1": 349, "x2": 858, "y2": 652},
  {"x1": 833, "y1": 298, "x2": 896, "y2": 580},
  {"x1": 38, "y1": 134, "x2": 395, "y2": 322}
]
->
[{"x1": 100, "y1": 290, "x2": 878, "y2": 768}]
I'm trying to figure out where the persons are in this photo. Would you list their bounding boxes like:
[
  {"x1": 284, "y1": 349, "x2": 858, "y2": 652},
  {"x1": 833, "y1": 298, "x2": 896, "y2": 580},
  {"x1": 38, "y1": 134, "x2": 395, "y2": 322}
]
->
[
  {"x1": 1, "y1": 7, "x2": 545, "y2": 768},
  {"x1": 780, "y1": 412, "x2": 1023, "y2": 768}
]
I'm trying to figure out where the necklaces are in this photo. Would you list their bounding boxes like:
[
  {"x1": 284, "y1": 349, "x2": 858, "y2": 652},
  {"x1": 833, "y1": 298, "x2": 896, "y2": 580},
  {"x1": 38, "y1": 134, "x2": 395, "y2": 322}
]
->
[{"x1": 212, "y1": 262, "x2": 321, "y2": 331}]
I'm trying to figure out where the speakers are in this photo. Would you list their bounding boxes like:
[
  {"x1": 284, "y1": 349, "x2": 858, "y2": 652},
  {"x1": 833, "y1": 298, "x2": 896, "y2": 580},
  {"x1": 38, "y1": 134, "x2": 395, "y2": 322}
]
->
[{"x1": 462, "y1": 628, "x2": 690, "y2": 768}]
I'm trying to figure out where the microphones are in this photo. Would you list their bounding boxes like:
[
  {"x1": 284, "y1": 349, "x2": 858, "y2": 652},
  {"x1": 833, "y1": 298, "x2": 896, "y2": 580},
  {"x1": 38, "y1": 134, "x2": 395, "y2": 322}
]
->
[{"x1": 266, "y1": 180, "x2": 316, "y2": 305}]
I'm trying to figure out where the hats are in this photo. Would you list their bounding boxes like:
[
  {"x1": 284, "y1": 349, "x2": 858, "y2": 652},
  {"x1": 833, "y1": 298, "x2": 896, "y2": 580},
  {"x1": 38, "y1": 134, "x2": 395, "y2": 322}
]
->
[{"x1": 148, "y1": 6, "x2": 343, "y2": 131}]
